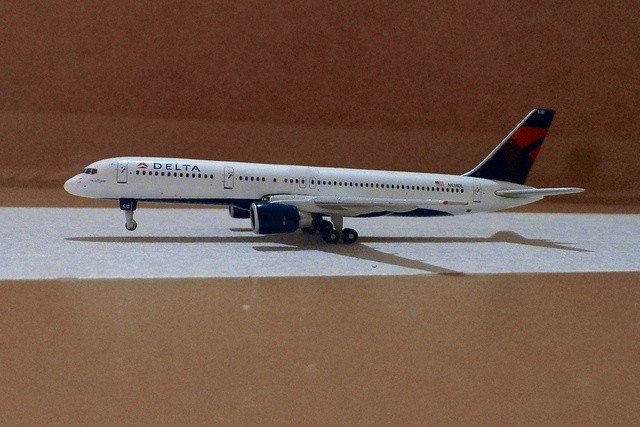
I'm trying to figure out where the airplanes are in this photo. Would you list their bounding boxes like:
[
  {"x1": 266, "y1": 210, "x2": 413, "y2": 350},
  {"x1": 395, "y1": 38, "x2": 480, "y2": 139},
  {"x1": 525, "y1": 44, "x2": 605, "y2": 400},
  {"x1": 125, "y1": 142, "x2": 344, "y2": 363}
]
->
[{"x1": 63, "y1": 108, "x2": 585, "y2": 244}]
